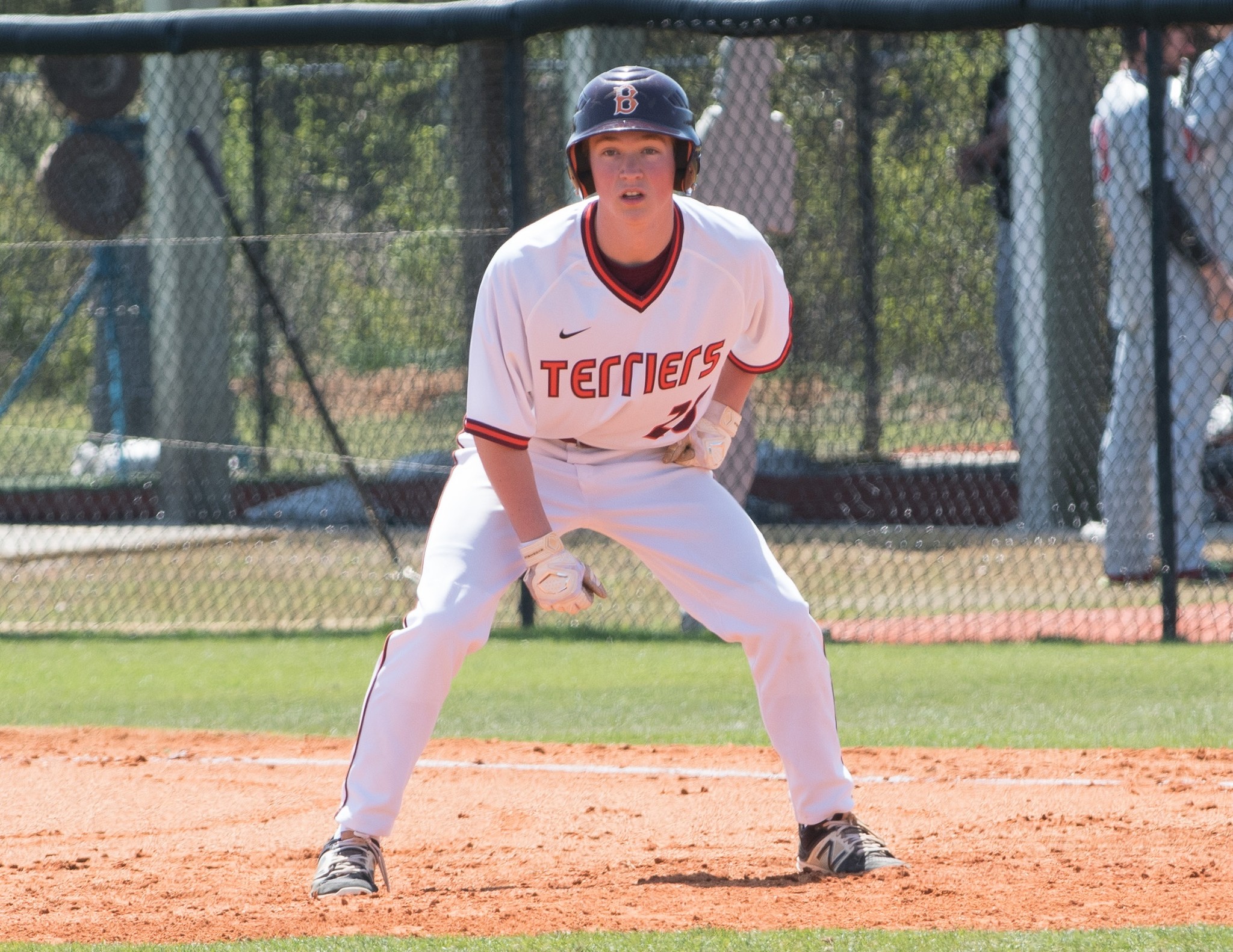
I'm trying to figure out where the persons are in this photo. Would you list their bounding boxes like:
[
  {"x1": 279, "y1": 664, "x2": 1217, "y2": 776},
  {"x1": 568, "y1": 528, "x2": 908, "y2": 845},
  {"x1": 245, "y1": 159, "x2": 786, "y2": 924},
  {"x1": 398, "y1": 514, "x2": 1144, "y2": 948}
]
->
[
  {"x1": 314, "y1": 66, "x2": 906, "y2": 895},
  {"x1": 955, "y1": 58, "x2": 1018, "y2": 449},
  {"x1": 1090, "y1": 0, "x2": 1233, "y2": 588}
]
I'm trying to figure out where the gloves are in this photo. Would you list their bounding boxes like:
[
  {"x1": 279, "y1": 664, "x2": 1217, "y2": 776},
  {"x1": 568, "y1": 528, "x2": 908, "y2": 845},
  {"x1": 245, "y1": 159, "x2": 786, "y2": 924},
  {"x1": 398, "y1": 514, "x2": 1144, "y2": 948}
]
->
[
  {"x1": 518, "y1": 531, "x2": 608, "y2": 615},
  {"x1": 662, "y1": 400, "x2": 742, "y2": 469}
]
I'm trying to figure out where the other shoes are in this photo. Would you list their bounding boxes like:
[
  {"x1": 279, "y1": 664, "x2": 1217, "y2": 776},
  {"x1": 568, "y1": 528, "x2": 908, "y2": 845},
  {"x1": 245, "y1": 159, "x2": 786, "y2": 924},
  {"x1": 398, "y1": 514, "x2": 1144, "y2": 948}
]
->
[
  {"x1": 1105, "y1": 560, "x2": 1162, "y2": 581},
  {"x1": 1178, "y1": 560, "x2": 1232, "y2": 584}
]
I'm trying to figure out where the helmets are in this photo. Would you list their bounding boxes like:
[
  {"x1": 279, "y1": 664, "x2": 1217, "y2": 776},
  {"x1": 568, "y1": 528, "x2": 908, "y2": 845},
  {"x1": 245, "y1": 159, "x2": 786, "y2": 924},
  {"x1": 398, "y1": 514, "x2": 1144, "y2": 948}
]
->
[{"x1": 565, "y1": 66, "x2": 702, "y2": 200}]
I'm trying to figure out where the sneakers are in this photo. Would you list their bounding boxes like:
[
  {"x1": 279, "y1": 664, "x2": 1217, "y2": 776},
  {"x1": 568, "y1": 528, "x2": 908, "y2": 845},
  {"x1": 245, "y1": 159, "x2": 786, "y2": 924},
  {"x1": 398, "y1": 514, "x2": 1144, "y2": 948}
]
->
[
  {"x1": 797, "y1": 812, "x2": 912, "y2": 878},
  {"x1": 311, "y1": 832, "x2": 389, "y2": 900}
]
